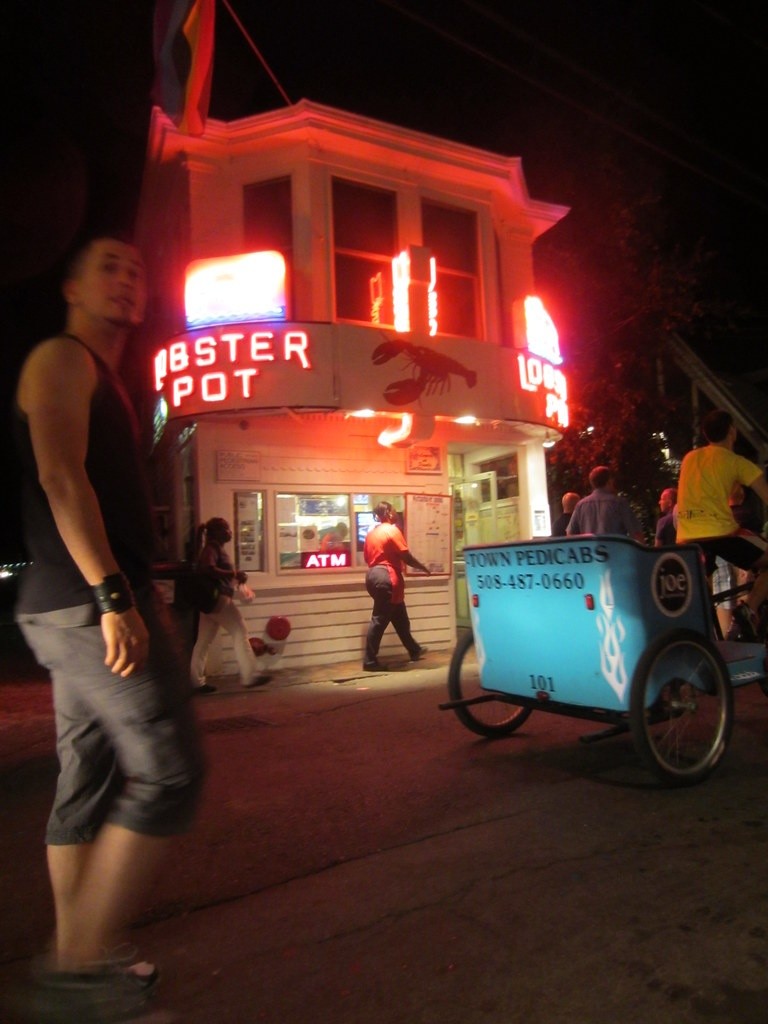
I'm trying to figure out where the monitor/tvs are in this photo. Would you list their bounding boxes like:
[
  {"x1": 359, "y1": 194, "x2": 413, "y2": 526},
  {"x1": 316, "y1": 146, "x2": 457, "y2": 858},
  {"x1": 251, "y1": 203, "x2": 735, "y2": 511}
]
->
[
  {"x1": 356, "y1": 512, "x2": 403, "y2": 554},
  {"x1": 184, "y1": 249, "x2": 287, "y2": 331}
]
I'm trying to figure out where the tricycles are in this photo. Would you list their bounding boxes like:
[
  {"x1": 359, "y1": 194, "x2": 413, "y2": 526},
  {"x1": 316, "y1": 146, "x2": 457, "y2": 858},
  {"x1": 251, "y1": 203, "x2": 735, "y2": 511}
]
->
[{"x1": 445, "y1": 514, "x2": 768, "y2": 788}]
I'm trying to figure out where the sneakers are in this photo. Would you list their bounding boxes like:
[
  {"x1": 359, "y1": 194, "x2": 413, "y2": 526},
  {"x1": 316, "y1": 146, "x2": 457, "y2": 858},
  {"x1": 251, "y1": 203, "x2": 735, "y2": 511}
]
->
[{"x1": 734, "y1": 604, "x2": 759, "y2": 640}]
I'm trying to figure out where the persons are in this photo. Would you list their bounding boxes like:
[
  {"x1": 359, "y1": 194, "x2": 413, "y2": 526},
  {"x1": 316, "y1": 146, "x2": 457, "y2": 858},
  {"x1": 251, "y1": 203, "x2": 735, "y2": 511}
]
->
[
  {"x1": 3, "y1": 232, "x2": 207, "y2": 1017},
  {"x1": 319, "y1": 523, "x2": 351, "y2": 555},
  {"x1": 186, "y1": 517, "x2": 273, "y2": 694},
  {"x1": 565, "y1": 465, "x2": 647, "y2": 546},
  {"x1": 550, "y1": 493, "x2": 582, "y2": 538},
  {"x1": 362, "y1": 501, "x2": 431, "y2": 671},
  {"x1": 653, "y1": 488, "x2": 678, "y2": 548},
  {"x1": 710, "y1": 482, "x2": 764, "y2": 642},
  {"x1": 673, "y1": 410, "x2": 768, "y2": 643}
]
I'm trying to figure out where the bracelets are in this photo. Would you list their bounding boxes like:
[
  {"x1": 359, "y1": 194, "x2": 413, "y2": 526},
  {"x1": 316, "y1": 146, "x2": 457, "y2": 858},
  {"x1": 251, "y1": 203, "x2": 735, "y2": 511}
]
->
[
  {"x1": 233, "y1": 568, "x2": 236, "y2": 580},
  {"x1": 88, "y1": 572, "x2": 139, "y2": 615}
]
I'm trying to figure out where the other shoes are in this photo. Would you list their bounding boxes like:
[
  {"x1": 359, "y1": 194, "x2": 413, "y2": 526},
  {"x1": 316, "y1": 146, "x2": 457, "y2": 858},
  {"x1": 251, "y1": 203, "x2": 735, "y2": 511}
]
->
[
  {"x1": 412, "y1": 645, "x2": 428, "y2": 661},
  {"x1": 194, "y1": 683, "x2": 219, "y2": 696},
  {"x1": 244, "y1": 675, "x2": 272, "y2": 689},
  {"x1": 41, "y1": 962, "x2": 157, "y2": 990},
  {"x1": 363, "y1": 663, "x2": 389, "y2": 671}
]
